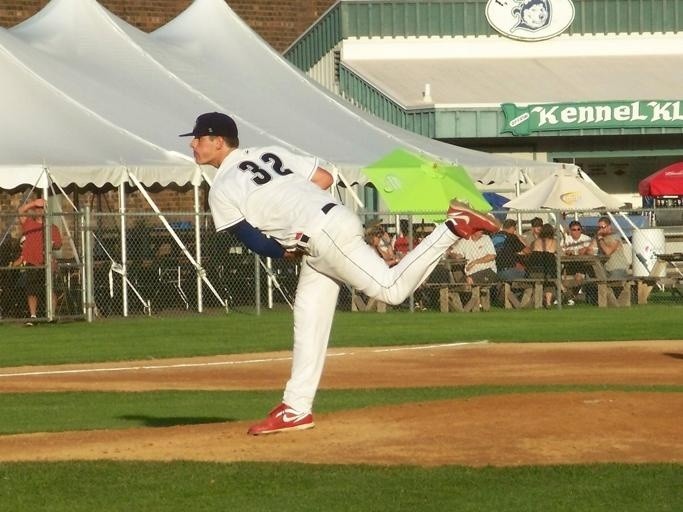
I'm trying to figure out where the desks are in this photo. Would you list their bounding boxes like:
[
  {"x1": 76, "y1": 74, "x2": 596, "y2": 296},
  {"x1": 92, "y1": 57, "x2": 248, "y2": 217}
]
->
[
  {"x1": 438, "y1": 259, "x2": 468, "y2": 310},
  {"x1": 561, "y1": 255, "x2": 619, "y2": 306},
  {"x1": 656, "y1": 253, "x2": 683, "y2": 260}
]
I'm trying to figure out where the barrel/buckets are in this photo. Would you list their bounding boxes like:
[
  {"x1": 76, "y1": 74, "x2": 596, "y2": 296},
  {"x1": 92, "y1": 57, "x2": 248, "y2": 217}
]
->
[{"x1": 631, "y1": 227, "x2": 667, "y2": 278}]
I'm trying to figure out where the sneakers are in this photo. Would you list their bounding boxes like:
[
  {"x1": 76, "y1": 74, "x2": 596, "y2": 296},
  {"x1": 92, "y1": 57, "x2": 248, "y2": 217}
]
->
[
  {"x1": 248, "y1": 402, "x2": 315, "y2": 436},
  {"x1": 445, "y1": 198, "x2": 500, "y2": 240},
  {"x1": 26, "y1": 317, "x2": 40, "y2": 326}
]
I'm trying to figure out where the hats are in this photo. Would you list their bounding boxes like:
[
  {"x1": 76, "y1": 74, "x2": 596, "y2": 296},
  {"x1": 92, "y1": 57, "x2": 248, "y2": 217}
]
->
[{"x1": 178, "y1": 112, "x2": 238, "y2": 137}]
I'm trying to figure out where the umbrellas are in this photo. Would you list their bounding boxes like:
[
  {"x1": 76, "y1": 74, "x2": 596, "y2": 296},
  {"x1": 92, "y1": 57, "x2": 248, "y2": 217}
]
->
[{"x1": 502, "y1": 164, "x2": 625, "y2": 310}]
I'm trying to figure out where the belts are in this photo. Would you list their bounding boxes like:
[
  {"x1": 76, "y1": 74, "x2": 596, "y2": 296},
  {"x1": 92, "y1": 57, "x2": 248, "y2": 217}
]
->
[{"x1": 296, "y1": 203, "x2": 337, "y2": 253}]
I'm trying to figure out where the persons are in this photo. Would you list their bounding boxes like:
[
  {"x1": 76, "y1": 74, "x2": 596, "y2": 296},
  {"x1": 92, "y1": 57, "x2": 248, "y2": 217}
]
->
[
  {"x1": 8, "y1": 198, "x2": 62, "y2": 326},
  {"x1": 362, "y1": 216, "x2": 629, "y2": 312},
  {"x1": 179, "y1": 112, "x2": 499, "y2": 437}
]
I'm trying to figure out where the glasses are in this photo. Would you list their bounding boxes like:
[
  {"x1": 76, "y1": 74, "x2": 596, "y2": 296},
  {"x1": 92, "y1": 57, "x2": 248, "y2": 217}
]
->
[
  {"x1": 572, "y1": 228, "x2": 580, "y2": 231},
  {"x1": 598, "y1": 225, "x2": 609, "y2": 229}
]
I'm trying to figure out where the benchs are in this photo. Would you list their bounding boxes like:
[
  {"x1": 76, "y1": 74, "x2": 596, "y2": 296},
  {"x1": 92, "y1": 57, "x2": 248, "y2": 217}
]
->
[{"x1": 351, "y1": 276, "x2": 682, "y2": 313}]
